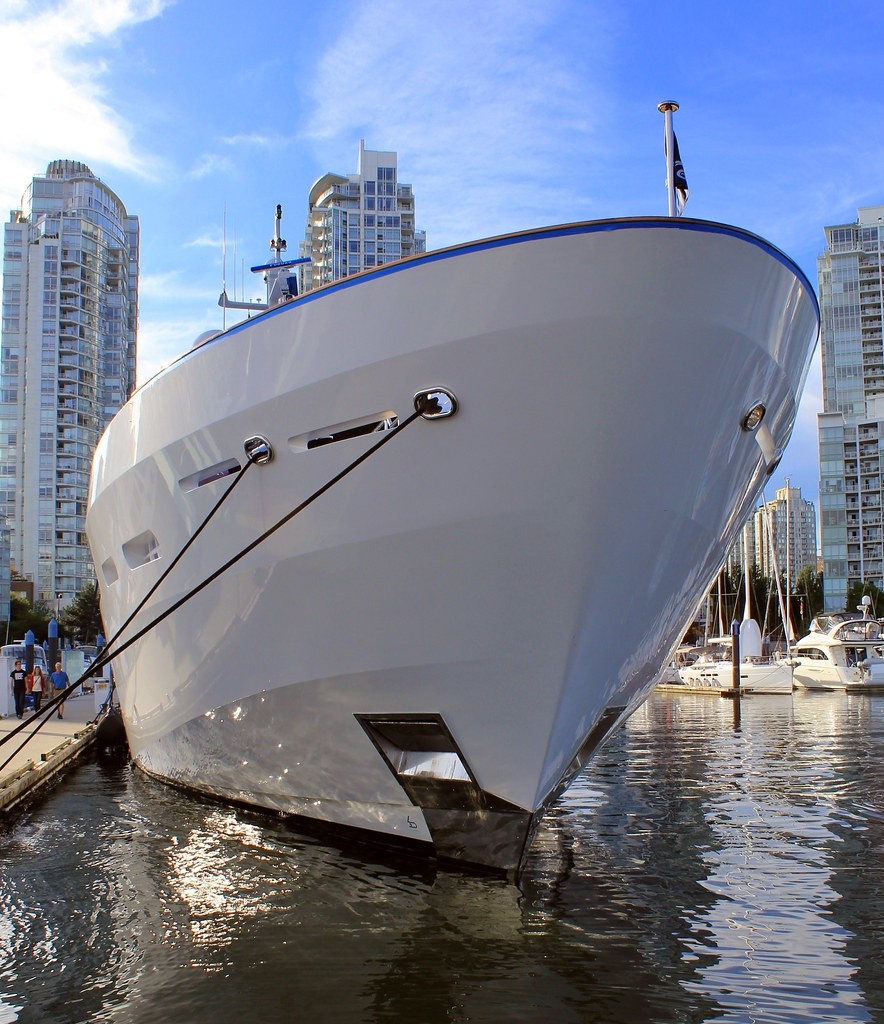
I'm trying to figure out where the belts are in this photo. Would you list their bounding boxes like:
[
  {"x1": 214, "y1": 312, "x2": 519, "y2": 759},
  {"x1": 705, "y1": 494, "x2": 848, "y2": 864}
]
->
[{"x1": 55, "y1": 688, "x2": 64, "y2": 690}]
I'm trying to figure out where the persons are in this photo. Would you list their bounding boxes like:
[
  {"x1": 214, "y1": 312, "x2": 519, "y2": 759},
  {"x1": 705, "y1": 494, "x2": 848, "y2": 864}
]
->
[
  {"x1": 809, "y1": 616, "x2": 827, "y2": 635},
  {"x1": 678, "y1": 652, "x2": 684, "y2": 668},
  {"x1": 50, "y1": 662, "x2": 70, "y2": 719},
  {"x1": 10, "y1": 660, "x2": 45, "y2": 719}
]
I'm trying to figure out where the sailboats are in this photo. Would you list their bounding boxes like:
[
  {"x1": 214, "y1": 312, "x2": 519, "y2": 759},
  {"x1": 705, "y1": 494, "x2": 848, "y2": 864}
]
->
[{"x1": 657, "y1": 474, "x2": 884, "y2": 693}]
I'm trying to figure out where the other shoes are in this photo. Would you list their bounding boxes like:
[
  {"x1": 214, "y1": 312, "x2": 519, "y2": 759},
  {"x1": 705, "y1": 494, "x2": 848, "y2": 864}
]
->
[
  {"x1": 17, "y1": 714, "x2": 22, "y2": 719},
  {"x1": 57, "y1": 713, "x2": 63, "y2": 719}
]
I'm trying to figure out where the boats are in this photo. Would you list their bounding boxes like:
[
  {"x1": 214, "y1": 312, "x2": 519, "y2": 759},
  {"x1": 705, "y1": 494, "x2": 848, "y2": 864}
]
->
[{"x1": 84, "y1": 200, "x2": 827, "y2": 881}]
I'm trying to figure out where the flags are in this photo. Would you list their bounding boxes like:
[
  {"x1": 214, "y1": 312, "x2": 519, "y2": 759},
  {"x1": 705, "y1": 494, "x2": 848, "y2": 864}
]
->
[{"x1": 663, "y1": 128, "x2": 689, "y2": 217}]
